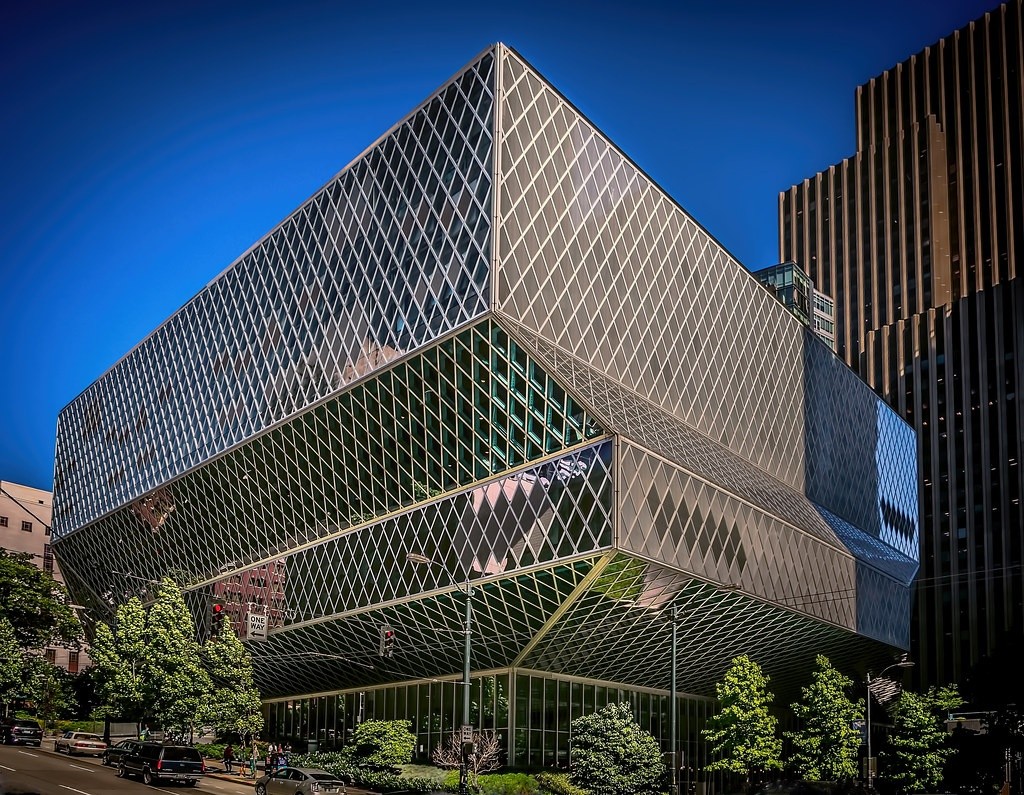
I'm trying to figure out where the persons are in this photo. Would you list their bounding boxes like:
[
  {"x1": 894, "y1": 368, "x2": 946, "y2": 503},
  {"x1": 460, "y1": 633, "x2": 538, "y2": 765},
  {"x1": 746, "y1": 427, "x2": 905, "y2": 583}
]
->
[
  {"x1": 235, "y1": 746, "x2": 247, "y2": 776},
  {"x1": 224, "y1": 745, "x2": 234, "y2": 772},
  {"x1": 141, "y1": 724, "x2": 150, "y2": 740},
  {"x1": 249, "y1": 746, "x2": 259, "y2": 777},
  {"x1": 268, "y1": 742, "x2": 292, "y2": 754}
]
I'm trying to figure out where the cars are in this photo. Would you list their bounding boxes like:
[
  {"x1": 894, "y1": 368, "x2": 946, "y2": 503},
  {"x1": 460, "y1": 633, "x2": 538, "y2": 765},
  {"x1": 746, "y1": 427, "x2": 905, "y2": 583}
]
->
[
  {"x1": 253, "y1": 767, "x2": 346, "y2": 795},
  {"x1": 54, "y1": 731, "x2": 108, "y2": 756}
]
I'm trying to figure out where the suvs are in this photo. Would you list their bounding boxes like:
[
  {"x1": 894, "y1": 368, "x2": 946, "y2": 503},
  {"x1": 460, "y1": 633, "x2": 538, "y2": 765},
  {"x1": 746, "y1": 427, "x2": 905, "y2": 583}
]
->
[
  {"x1": 118, "y1": 742, "x2": 206, "y2": 787},
  {"x1": 103, "y1": 739, "x2": 143, "y2": 766},
  {"x1": 0, "y1": 718, "x2": 43, "y2": 747}
]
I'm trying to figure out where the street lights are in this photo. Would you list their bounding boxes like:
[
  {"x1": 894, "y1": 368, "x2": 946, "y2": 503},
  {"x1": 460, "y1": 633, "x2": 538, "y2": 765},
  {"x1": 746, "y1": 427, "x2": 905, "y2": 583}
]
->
[
  {"x1": 404, "y1": 551, "x2": 476, "y2": 795},
  {"x1": 865, "y1": 661, "x2": 917, "y2": 795},
  {"x1": 664, "y1": 582, "x2": 743, "y2": 794}
]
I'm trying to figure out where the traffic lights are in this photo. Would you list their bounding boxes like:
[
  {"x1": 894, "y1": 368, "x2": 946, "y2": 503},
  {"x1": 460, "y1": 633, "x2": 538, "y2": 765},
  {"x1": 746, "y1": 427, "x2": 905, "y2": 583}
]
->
[
  {"x1": 212, "y1": 603, "x2": 224, "y2": 633},
  {"x1": 380, "y1": 625, "x2": 395, "y2": 658}
]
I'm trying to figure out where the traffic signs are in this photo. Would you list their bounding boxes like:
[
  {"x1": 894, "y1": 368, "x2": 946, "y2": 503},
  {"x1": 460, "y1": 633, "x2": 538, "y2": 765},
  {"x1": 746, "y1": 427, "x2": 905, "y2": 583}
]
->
[
  {"x1": 247, "y1": 613, "x2": 268, "y2": 642},
  {"x1": 462, "y1": 726, "x2": 473, "y2": 743}
]
